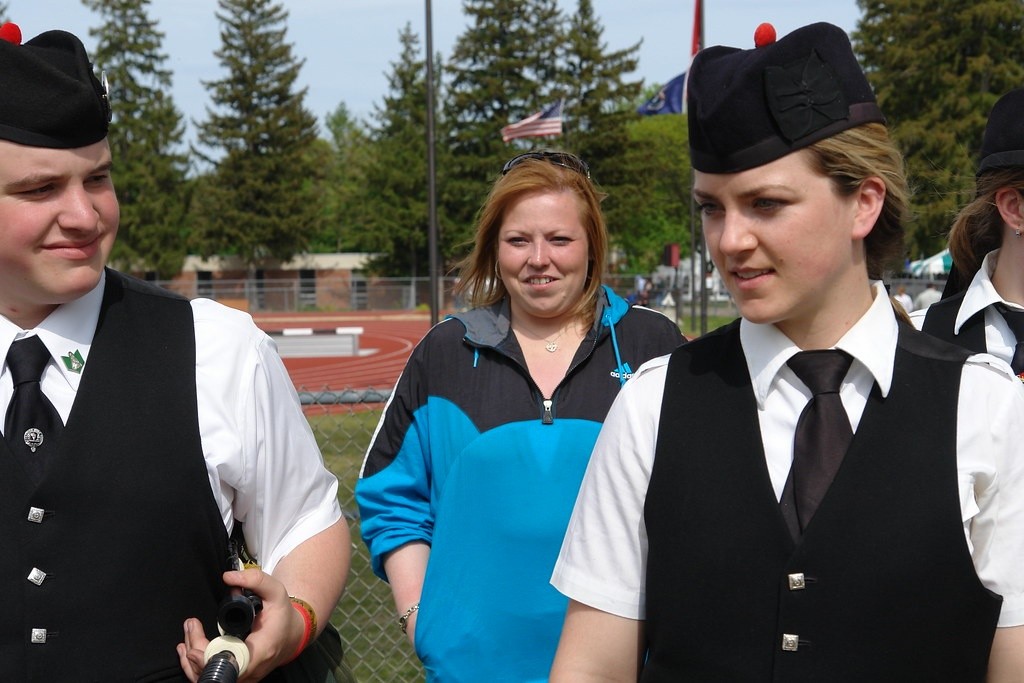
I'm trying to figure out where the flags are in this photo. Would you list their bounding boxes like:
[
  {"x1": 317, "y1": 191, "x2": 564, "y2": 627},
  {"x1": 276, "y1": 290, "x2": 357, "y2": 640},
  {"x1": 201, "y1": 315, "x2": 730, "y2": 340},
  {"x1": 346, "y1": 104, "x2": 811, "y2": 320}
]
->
[
  {"x1": 629, "y1": 69, "x2": 688, "y2": 115},
  {"x1": 500, "y1": 100, "x2": 563, "y2": 141}
]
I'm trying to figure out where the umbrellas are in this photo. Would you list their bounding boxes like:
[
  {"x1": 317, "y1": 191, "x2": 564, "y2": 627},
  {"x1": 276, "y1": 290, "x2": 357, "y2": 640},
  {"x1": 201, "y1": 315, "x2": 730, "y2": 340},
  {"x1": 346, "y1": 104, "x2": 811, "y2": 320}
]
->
[{"x1": 903, "y1": 246, "x2": 953, "y2": 276}]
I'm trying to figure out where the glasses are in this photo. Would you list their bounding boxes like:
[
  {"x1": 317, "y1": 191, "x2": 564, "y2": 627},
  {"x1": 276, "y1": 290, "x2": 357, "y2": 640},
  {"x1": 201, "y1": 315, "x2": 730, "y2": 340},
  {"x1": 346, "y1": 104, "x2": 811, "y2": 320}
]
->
[{"x1": 501, "y1": 150, "x2": 591, "y2": 180}]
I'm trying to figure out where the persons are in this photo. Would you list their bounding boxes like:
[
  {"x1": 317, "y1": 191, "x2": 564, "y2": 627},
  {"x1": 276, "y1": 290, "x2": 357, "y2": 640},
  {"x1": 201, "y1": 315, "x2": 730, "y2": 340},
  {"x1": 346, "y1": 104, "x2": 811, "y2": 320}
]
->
[
  {"x1": 545, "y1": 22, "x2": 1024, "y2": 683},
  {"x1": 354, "y1": 151, "x2": 693, "y2": 683},
  {"x1": 0, "y1": 30, "x2": 350, "y2": 682}
]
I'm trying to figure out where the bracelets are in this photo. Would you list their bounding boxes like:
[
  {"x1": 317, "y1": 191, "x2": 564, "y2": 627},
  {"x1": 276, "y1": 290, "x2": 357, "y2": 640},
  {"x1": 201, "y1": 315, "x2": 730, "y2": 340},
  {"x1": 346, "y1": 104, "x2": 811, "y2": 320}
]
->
[
  {"x1": 290, "y1": 597, "x2": 317, "y2": 660},
  {"x1": 398, "y1": 603, "x2": 418, "y2": 634}
]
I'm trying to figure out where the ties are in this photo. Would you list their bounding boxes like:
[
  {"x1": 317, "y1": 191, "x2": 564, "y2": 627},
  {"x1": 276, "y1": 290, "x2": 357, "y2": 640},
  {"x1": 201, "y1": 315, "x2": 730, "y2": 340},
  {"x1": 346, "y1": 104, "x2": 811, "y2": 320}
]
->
[
  {"x1": 2, "y1": 333, "x2": 65, "y2": 486},
  {"x1": 990, "y1": 302, "x2": 1024, "y2": 382},
  {"x1": 779, "y1": 348, "x2": 857, "y2": 543}
]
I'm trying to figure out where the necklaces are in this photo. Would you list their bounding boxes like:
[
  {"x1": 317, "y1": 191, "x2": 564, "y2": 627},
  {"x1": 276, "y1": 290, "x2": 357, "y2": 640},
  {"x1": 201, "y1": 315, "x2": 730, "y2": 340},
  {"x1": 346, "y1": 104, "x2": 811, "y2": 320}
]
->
[{"x1": 514, "y1": 320, "x2": 576, "y2": 352}]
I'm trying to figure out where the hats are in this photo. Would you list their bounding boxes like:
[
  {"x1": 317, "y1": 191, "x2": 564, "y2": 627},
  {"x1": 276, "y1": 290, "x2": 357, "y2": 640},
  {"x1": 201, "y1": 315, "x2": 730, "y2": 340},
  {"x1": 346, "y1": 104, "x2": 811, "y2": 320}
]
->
[
  {"x1": 684, "y1": 19, "x2": 889, "y2": 175},
  {"x1": 970, "y1": 87, "x2": 1024, "y2": 178},
  {"x1": 0, "y1": 21, "x2": 114, "y2": 148}
]
